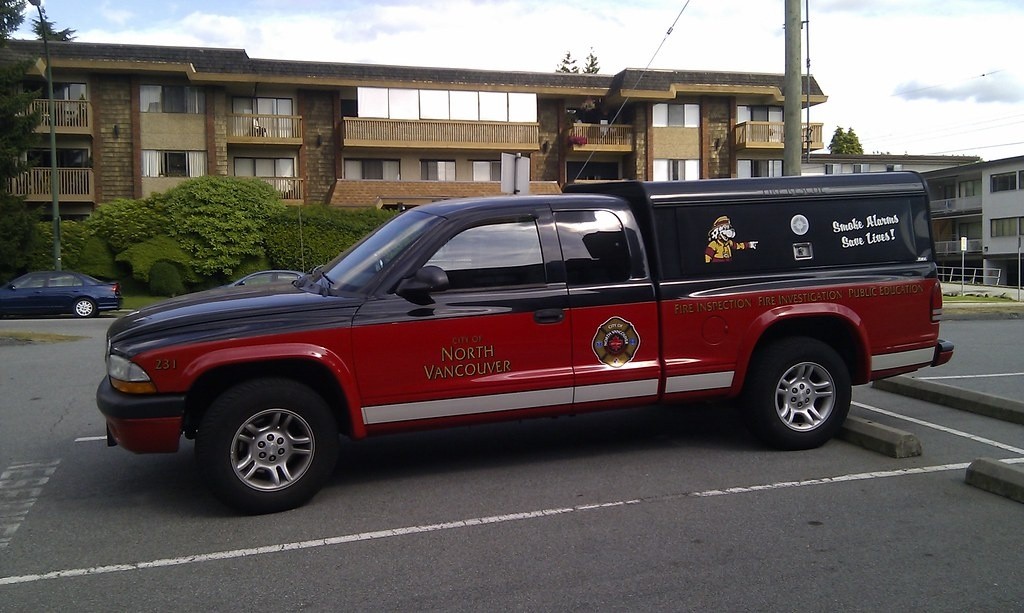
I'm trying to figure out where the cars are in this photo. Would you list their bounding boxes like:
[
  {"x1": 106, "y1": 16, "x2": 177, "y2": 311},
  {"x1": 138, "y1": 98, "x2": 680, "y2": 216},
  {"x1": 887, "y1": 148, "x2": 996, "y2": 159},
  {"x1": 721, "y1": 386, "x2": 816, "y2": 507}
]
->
[
  {"x1": 0, "y1": 270, "x2": 123, "y2": 320},
  {"x1": 213, "y1": 270, "x2": 306, "y2": 287}
]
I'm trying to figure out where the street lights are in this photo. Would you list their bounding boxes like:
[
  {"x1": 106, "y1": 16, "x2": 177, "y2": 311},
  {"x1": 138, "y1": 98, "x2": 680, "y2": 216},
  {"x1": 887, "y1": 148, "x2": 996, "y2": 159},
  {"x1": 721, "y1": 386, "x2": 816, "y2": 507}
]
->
[{"x1": 27, "y1": 0, "x2": 61, "y2": 274}]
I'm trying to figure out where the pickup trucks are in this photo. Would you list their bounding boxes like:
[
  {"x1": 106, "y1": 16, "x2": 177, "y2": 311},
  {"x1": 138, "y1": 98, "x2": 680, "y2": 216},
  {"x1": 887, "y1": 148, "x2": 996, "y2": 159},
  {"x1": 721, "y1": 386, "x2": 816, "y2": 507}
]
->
[{"x1": 96, "y1": 171, "x2": 953, "y2": 514}]
